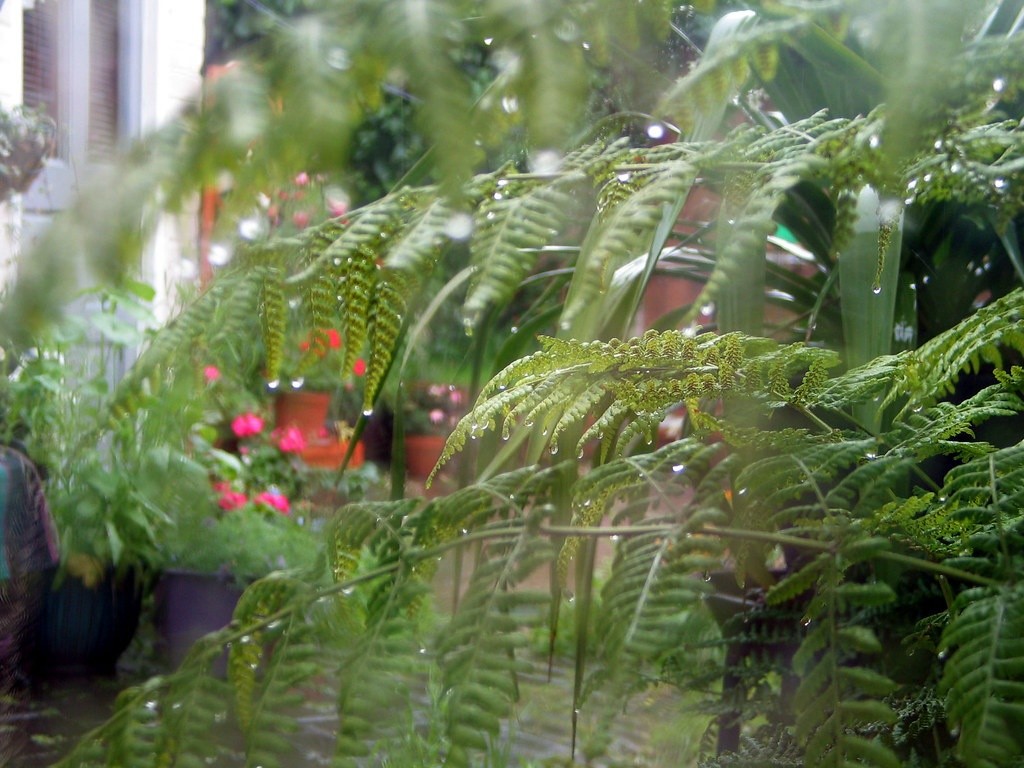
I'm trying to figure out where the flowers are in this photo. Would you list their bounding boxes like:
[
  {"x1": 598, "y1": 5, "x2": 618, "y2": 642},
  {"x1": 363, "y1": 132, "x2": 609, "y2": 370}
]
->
[
  {"x1": 407, "y1": 377, "x2": 468, "y2": 429},
  {"x1": 203, "y1": 380, "x2": 298, "y2": 526},
  {"x1": 299, "y1": 327, "x2": 371, "y2": 382}
]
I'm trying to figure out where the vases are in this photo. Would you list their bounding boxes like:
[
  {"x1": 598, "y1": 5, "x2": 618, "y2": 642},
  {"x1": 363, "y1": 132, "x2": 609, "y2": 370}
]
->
[
  {"x1": 402, "y1": 429, "x2": 451, "y2": 484},
  {"x1": 276, "y1": 385, "x2": 333, "y2": 434},
  {"x1": 162, "y1": 564, "x2": 300, "y2": 690}
]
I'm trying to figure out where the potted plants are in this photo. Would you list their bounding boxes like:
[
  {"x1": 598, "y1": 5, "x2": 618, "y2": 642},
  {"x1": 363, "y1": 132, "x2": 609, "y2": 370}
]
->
[{"x1": 299, "y1": 423, "x2": 365, "y2": 475}]
